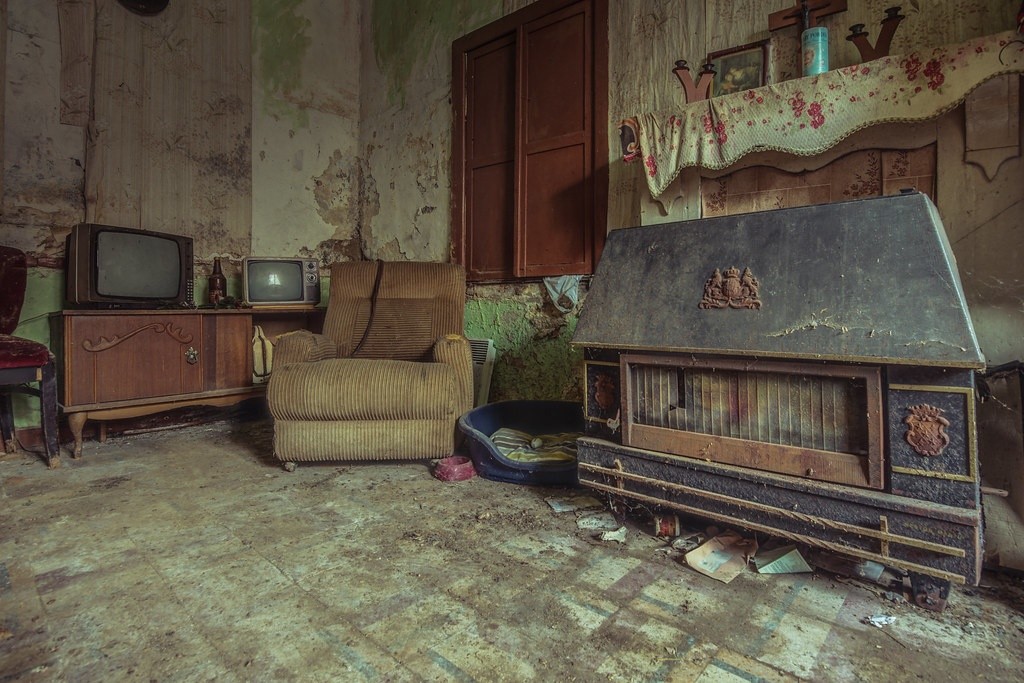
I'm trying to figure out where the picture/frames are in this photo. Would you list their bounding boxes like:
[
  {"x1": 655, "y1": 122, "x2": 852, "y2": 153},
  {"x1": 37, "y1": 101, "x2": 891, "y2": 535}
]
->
[{"x1": 706, "y1": 39, "x2": 767, "y2": 100}]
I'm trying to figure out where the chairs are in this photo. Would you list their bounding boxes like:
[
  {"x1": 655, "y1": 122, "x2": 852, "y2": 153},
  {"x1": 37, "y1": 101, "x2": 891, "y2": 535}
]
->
[{"x1": 0, "y1": 247, "x2": 62, "y2": 468}]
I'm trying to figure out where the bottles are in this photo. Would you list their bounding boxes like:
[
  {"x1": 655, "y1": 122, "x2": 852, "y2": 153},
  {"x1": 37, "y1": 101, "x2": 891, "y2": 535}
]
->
[{"x1": 209, "y1": 257, "x2": 227, "y2": 305}]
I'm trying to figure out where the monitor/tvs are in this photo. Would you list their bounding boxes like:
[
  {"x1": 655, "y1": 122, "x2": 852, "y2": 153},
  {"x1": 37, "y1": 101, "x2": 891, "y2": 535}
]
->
[
  {"x1": 242, "y1": 257, "x2": 321, "y2": 309},
  {"x1": 65, "y1": 223, "x2": 194, "y2": 309}
]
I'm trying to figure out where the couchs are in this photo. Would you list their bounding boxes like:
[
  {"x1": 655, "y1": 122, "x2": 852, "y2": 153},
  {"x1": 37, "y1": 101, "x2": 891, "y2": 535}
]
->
[{"x1": 270, "y1": 263, "x2": 474, "y2": 474}]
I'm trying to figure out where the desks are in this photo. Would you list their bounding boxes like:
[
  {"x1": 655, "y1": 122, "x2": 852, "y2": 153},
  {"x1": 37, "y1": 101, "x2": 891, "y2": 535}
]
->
[{"x1": 50, "y1": 308, "x2": 326, "y2": 459}]
